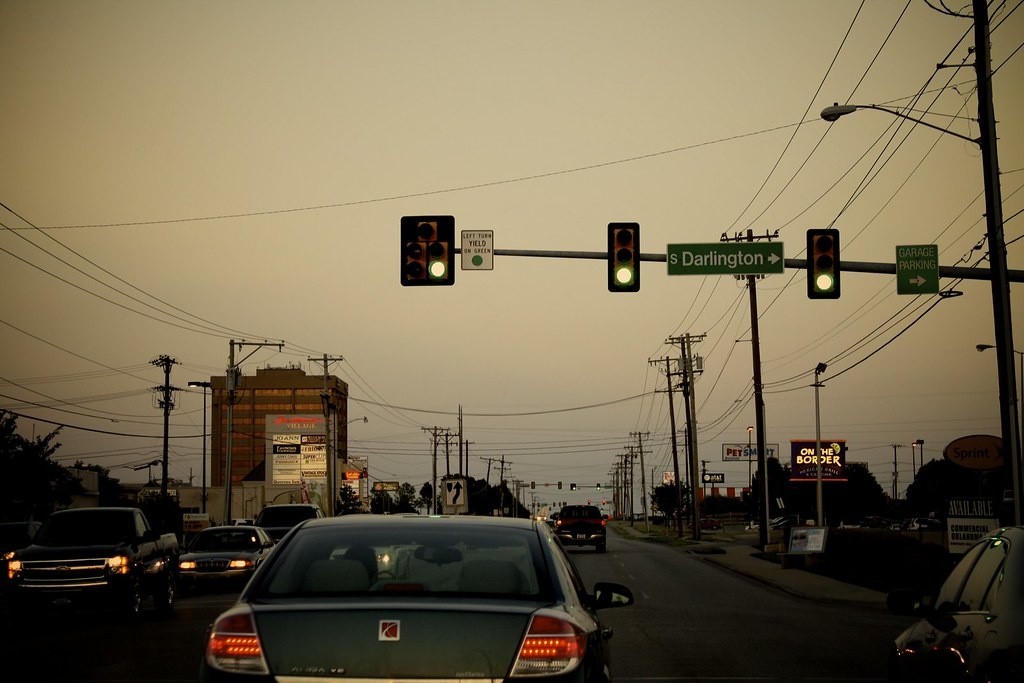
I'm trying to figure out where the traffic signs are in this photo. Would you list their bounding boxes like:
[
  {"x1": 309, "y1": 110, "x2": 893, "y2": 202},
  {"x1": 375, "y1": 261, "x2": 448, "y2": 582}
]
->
[
  {"x1": 667, "y1": 243, "x2": 785, "y2": 274},
  {"x1": 896, "y1": 246, "x2": 939, "y2": 294}
]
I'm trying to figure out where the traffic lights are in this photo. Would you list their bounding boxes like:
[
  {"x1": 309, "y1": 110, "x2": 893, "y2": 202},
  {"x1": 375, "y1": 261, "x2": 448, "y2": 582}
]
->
[
  {"x1": 596, "y1": 483, "x2": 600, "y2": 490},
  {"x1": 607, "y1": 222, "x2": 639, "y2": 292},
  {"x1": 806, "y1": 228, "x2": 841, "y2": 299},
  {"x1": 588, "y1": 500, "x2": 591, "y2": 504},
  {"x1": 603, "y1": 500, "x2": 605, "y2": 504},
  {"x1": 401, "y1": 215, "x2": 456, "y2": 287},
  {"x1": 571, "y1": 483, "x2": 576, "y2": 490}
]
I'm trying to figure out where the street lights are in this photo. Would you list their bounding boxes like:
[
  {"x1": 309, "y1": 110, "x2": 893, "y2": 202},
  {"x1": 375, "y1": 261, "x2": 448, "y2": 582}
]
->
[
  {"x1": 820, "y1": 104, "x2": 1024, "y2": 523},
  {"x1": 325, "y1": 416, "x2": 368, "y2": 517},
  {"x1": 747, "y1": 426, "x2": 754, "y2": 499},
  {"x1": 188, "y1": 381, "x2": 207, "y2": 514},
  {"x1": 976, "y1": 345, "x2": 1023, "y2": 497},
  {"x1": 814, "y1": 363, "x2": 828, "y2": 525},
  {"x1": 911, "y1": 440, "x2": 925, "y2": 479}
]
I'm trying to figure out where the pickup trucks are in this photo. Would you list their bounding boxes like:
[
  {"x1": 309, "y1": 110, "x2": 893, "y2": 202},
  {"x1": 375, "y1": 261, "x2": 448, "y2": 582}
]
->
[
  {"x1": 0, "y1": 506, "x2": 180, "y2": 620},
  {"x1": 256, "y1": 503, "x2": 327, "y2": 544},
  {"x1": 554, "y1": 505, "x2": 609, "y2": 552}
]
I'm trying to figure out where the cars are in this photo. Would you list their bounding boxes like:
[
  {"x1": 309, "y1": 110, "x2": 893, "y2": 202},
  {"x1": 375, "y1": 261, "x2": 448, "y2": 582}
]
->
[
  {"x1": 887, "y1": 523, "x2": 1023, "y2": 683},
  {"x1": 745, "y1": 515, "x2": 941, "y2": 531},
  {"x1": 688, "y1": 519, "x2": 722, "y2": 530},
  {"x1": 205, "y1": 513, "x2": 633, "y2": 683},
  {"x1": 179, "y1": 524, "x2": 280, "y2": 588},
  {"x1": 0, "y1": 521, "x2": 42, "y2": 555},
  {"x1": 330, "y1": 542, "x2": 410, "y2": 580}
]
